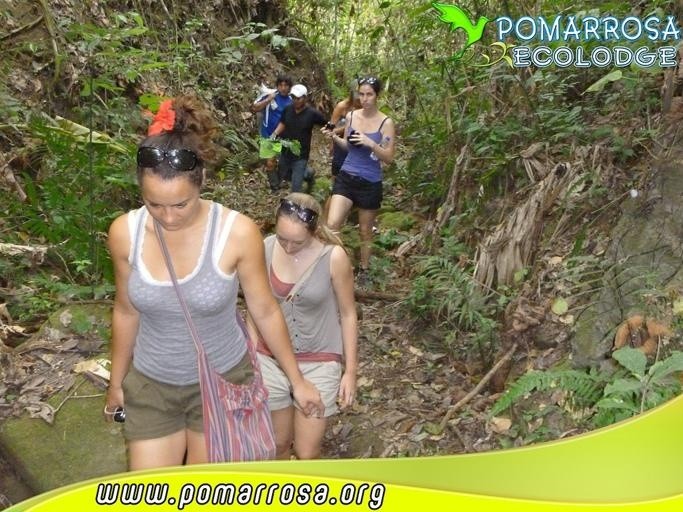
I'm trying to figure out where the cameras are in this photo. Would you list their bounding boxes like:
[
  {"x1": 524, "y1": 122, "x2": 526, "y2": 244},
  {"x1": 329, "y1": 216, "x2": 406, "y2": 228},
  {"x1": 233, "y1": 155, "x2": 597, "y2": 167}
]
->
[
  {"x1": 114, "y1": 408, "x2": 126, "y2": 423},
  {"x1": 326, "y1": 122, "x2": 336, "y2": 131},
  {"x1": 347, "y1": 130, "x2": 363, "y2": 149}
]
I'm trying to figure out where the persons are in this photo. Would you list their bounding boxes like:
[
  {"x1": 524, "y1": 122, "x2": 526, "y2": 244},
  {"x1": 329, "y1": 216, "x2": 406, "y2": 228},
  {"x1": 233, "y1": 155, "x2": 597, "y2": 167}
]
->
[
  {"x1": 102, "y1": 90, "x2": 327, "y2": 469},
  {"x1": 241, "y1": 188, "x2": 363, "y2": 461},
  {"x1": 323, "y1": 75, "x2": 396, "y2": 272},
  {"x1": 252, "y1": 75, "x2": 293, "y2": 192},
  {"x1": 267, "y1": 82, "x2": 328, "y2": 193},
  {"x1": 319, "y1": 79, "x2": 362, "y2": 215}
]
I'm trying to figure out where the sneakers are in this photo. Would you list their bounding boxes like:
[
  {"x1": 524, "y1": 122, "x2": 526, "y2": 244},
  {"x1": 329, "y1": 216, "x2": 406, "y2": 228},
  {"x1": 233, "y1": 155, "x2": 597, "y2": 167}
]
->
[{"x1": 305, "y1": 167, "x2": 315, "y2": 190}]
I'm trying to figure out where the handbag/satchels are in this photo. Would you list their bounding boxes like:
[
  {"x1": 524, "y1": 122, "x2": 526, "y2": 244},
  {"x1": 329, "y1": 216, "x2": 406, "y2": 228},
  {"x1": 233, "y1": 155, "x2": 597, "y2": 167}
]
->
[{"x1": 196, "y1": 337, "x2": 277, "y2": 463}]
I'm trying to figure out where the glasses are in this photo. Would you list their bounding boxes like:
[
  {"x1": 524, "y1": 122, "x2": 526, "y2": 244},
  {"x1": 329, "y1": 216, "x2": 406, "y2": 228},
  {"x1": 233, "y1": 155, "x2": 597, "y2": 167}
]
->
[
  {"x1": 137, "y1": 147, "x2": 201, "y2": 171},
  {"x1": 357, "y1": 78, "x2": 376, "y2": 88},
  {"x1": 279, "y1": 197, "x2": 319, "y2": 223}
]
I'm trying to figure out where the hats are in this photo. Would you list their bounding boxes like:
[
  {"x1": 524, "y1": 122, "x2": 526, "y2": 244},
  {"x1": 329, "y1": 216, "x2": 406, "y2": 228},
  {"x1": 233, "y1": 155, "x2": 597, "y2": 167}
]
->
[{"x1": 288, "y1": 84, "x2": 308, "y2": 98}]
